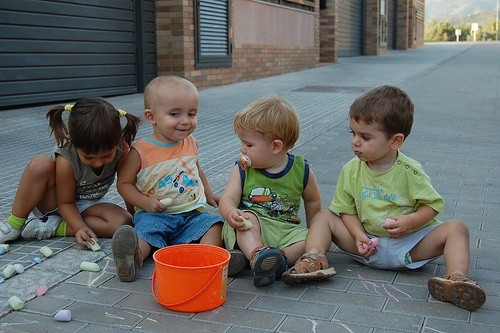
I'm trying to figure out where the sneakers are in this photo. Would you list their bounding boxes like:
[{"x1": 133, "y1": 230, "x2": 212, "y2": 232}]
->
[
  {"x1": 0, "y1": 220, "x2": 22, "y2": 243},
  {"x1": 21, "y1": 214, "x2": 63, "y2": 241}
]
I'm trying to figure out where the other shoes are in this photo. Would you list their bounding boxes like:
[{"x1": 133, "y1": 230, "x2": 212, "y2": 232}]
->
[
  {"x1": 228, "y1": 250, "x2": 245, "y2": 276},
  {"x1": 112, "y1": 225, "x2": 143, "y2": 282},
  {"x1": 250, "y1": 246, "x2": 286, "y2": 288}
]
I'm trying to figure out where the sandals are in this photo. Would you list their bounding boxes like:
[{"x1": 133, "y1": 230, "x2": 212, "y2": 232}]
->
[
  {"x1": 428, "y1": 274, "x2": 486, "y2": 311},
  {"x1": 282, "y1": 253, "x2": 336, "y2": 284}
]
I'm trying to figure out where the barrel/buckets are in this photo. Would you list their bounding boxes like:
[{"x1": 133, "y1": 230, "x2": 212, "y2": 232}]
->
[{"x1": 152, "y1": 244, "x2": 232, "y2": 312}]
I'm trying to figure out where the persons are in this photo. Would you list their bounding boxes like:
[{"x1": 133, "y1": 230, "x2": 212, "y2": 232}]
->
[
  {"x1": 112, "y1": 75, "x2": 247, "y2": 282},
  {"x1": 282, "y1": 85, "x2": 487, "y2": 311},
  {"x1": 219, "y1": 96, "x2": 321, "y2": 287},
  {"x1": 0, "y1": 98, "x2": 145, "y2": 250}
]
